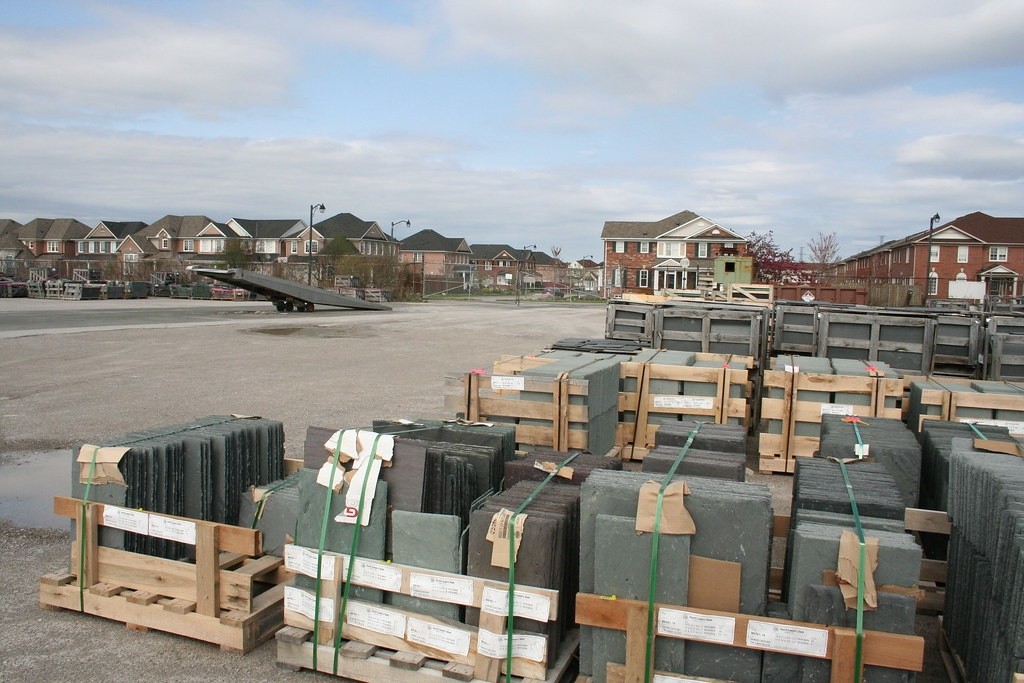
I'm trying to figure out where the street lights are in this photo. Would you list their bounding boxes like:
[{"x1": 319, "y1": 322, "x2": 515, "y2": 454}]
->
[
  {"x1": 923, "y1": 213, "x2": 941, "y2": 305},
  {"x1": 308, "y1": 202, "x2": 326, "y2": 287},
  {"x1": 524, "y1": 245, "x2": 536, "y2": 250},
  {"x1": 583, "y1": 255, "x2": 593, "y2": 266},
  {"x1": 390, "y1": 219, "x2": 412, "y2": 259}
]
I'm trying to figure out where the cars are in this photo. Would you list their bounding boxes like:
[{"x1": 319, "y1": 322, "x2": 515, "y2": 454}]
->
[
  {"x1": 236, "y1": 287, "x2": 251, "y2": 296},
  {"x1": 210, "y1": 284, "x2": 233, "y2": 296},
  {"x1": 543, "y1": 287, "x2": 564, "y2": 298}
]
